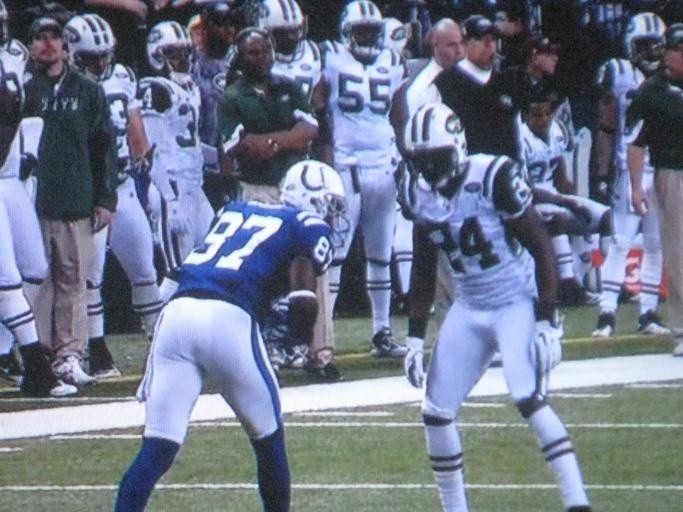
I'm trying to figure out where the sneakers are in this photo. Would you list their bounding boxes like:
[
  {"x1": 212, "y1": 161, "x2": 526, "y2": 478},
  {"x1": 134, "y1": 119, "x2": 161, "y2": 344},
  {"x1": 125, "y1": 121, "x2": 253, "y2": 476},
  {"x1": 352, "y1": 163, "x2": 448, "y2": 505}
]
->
[
  {"x1": 592, "y1": 311, "x2": 618, "y2": 339},
  {"x1": 285, "y1": 344, "x2": 310, "y2": 368},
  {"x1": 370, "y1": 328, "x2": 409, "y2": 357},
  {"x1": 638, "y1": 308, "x2": 673, "y2": 336},
  {"x1": 561, "y1": 276, "x2": 601, "y2": 305},
  {"x1": 306, "y1": 359, "x2": 343, "y2": 382},
  {"x1": 0, "y1": 337, "x2": 121, "y2": 396}
]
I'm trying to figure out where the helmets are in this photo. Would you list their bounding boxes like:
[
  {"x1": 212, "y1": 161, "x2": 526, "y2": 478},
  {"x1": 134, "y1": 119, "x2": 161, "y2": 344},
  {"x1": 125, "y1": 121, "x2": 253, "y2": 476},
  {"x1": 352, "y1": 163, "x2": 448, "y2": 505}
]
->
[
  {"x1": 404, "y1": 102, "x2": 469, "y2": 191},
  {"x1": 63, "y1": 13, "x2": 117, "y2": 86},
  {"x1": 339, "y1": 2, "x2": 387, "y2": 64},
  {"x1": 146, "y1": 19, "x2": 197, "y2": 92},
  {"x1": 257, "y1": 1, "x2": 309, "y2": 65},
  {"x1": 201, "y1": 1, "x2": 234, "y2": 23},
  {"x1": 626, "y1": 10, "x2": 667, "y2": 76},
  {"x1": 279, "y1": 159, "x2": 351, "y2": 250}
]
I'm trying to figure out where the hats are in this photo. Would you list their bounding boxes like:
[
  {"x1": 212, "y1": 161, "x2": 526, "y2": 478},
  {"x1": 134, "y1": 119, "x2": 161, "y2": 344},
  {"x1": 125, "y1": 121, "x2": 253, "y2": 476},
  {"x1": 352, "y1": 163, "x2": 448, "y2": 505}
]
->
[
  {"x1": 662, "y1": 22, "x2": 683, "y2": 47},
  {"x1": 522, "y1": 34, "x2": 564, "y2": 54},
  {"x1": 28, "y1": 16, "x2": 65, "y2": 37},
  {"x1": 463, "y1": 15, "x2": 501, "y2": 38}
]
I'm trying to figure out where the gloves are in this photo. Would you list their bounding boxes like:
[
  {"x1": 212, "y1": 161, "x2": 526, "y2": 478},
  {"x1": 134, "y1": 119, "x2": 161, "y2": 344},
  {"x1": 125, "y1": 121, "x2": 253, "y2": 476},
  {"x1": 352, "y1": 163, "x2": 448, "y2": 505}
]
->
[
  {"x1": 404, "y1": 335, "x2": 425, "y2": 388},
  {"x1": 528, "y1": 321, "x2": 562, "y2": 376}
]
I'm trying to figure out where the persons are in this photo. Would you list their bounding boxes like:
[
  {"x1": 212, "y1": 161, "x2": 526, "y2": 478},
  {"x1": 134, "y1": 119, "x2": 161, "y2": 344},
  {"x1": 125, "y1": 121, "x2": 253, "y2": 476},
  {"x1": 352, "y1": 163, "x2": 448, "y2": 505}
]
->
[
  {"x1": 1, "y1": 2, "x2": 682, "y2": 398},
  {"x1": 395, "y1": 104, "x2": 593, "y2": 511},
  {"x1": 114, "y1": 160, "x2": 352, "y2": 511}
]
